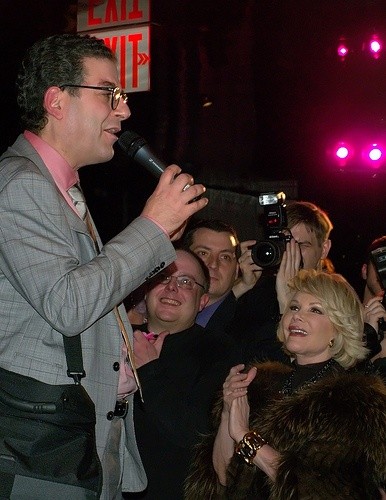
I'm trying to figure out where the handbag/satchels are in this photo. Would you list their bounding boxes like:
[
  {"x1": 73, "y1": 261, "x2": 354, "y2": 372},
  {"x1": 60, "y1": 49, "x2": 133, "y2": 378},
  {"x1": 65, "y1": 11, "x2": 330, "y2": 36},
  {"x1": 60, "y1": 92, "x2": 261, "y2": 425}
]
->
[{"x1": 0, "y1": 369, "x2": 104, "y2": 500}]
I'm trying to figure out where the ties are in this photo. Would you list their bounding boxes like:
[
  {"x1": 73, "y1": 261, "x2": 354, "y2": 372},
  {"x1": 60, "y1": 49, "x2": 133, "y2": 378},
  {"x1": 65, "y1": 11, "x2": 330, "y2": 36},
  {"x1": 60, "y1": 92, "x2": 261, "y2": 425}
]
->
[{"x1": 67, "y1": 184, "x2": 100, "y2": 255}]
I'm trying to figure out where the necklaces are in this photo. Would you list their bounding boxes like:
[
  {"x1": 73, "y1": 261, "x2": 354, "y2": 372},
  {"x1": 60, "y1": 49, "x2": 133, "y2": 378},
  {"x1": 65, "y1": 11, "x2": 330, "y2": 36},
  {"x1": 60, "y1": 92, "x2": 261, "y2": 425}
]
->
[{"x1": 281, "y1": 358, "x2": 337, "y2": 400}]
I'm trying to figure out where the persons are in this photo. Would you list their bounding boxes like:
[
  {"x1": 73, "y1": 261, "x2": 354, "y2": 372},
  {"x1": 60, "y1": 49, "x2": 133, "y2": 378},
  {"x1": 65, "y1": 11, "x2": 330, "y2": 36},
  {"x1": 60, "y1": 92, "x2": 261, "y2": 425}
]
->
[
  {"x1": 183, "y1": 268, "x2": 386, "y2": 500},
  {"x1": 0, "y1": 31, "x2": 209, "y2": 500},
  {"x1": 360, "y1": 236, "x2": 386, "y2": 372},
  {"x1": 183, "y1": 201, "x2": 334, "y2": 343},
  {"x1": 133, "y1": 247, "x2": 223, "y2": 500}
]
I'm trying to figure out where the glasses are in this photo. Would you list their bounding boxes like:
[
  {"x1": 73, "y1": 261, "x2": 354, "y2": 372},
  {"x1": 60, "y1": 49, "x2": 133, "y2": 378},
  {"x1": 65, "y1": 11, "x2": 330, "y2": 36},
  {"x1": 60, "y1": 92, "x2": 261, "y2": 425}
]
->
[
  {"x1": 59, "y1": 84, "x2": 127, "y2": 110},
  {"x1": 151, "y1": 272, "x2": 207, "y2": 293}
]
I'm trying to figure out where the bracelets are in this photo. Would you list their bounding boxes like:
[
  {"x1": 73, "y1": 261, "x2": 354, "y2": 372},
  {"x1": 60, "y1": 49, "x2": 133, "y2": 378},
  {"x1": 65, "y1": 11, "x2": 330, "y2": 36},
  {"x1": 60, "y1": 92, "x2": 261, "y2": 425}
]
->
[{"x1": 235, "y1": 430, "x2": 269, "y2": 466}]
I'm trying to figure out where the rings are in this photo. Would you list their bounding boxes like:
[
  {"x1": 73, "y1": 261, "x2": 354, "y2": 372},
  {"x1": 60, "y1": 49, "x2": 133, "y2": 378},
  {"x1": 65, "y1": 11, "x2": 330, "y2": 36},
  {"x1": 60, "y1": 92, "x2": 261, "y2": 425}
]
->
[{"x1": 363, "y1": 302, "x2": 370, "y2": 310}]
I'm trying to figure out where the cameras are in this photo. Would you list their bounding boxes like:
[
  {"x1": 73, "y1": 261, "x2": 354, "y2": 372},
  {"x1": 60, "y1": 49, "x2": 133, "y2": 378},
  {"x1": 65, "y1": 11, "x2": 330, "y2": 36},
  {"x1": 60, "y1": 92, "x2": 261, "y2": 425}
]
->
[{"x1": 250, "y1": 192, "x2": 293, "y2": 274}]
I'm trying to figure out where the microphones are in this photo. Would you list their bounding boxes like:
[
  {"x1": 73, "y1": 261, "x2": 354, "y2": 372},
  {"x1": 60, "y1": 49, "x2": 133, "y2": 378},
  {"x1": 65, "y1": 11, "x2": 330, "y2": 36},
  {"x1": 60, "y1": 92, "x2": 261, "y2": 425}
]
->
[{"x1": 118, "y1": 131, "x2": 199, "y2": 203}]
